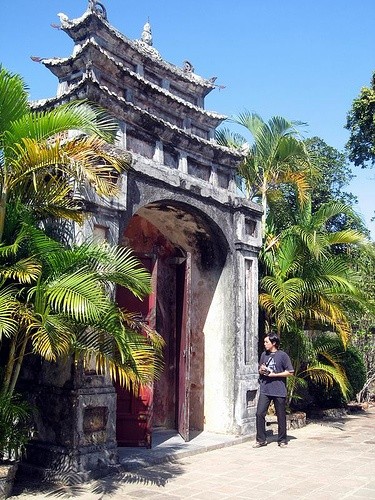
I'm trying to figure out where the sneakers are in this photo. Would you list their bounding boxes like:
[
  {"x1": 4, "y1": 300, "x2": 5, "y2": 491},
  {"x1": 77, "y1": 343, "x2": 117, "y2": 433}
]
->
[
  {"x1": 278, "y1": 442, "x2": 288, "y2": 448},
  {"x1": 252, "y1": 441, "x2": 268, "y2": 448}
]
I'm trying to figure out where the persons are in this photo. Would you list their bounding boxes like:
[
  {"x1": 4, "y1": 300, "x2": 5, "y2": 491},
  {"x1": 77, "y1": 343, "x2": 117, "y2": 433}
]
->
[{"x1": 253, "y1": 332, "x2": 294, "y2": 448}]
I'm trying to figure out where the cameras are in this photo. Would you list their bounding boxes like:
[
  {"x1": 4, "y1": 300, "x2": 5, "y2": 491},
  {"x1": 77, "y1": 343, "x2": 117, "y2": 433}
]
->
[{"x1": 262, "y1": 370, "x2": 269, "y2": 376}]
